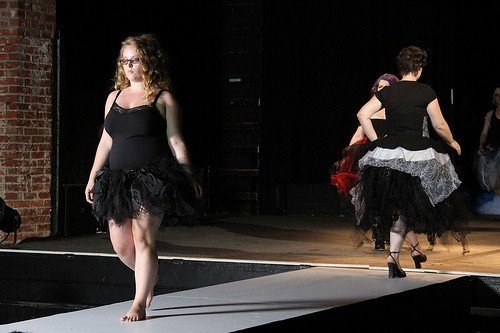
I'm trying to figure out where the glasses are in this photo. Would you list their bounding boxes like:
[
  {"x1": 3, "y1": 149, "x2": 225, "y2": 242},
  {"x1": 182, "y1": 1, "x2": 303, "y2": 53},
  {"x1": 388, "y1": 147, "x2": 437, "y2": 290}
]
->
[{"x1": 119, "y1": 58, "x2": 141, "y2": 65}]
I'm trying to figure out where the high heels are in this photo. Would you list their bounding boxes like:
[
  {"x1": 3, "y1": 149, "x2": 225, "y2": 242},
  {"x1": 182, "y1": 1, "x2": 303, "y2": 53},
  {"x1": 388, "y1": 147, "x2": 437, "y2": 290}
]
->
[
  {"x1": 410, "y1": 241, "x2": 427, "y2": 269},
  {"x1": 386, "y1": 252, "x2": 406, "y2": 278}
]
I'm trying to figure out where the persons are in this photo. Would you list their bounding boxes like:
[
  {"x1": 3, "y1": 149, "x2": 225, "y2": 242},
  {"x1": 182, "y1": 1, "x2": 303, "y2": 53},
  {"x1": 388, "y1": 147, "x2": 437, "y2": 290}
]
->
[
  {"x1": 478, "y1": 87, "x2": 500, "y2": 197},
  {"x1": 85, "y1": 34, "x2": 203, "y2": 322},
  {"x1": 331, "y1": 47, "x2": 461, "y2": 279}
]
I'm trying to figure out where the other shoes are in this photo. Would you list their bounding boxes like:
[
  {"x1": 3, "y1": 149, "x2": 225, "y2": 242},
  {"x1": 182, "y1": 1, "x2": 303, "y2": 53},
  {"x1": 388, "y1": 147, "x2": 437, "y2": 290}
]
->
[{"x1": 375, "y1": 235, "x2": 385, "y2": 250}]
